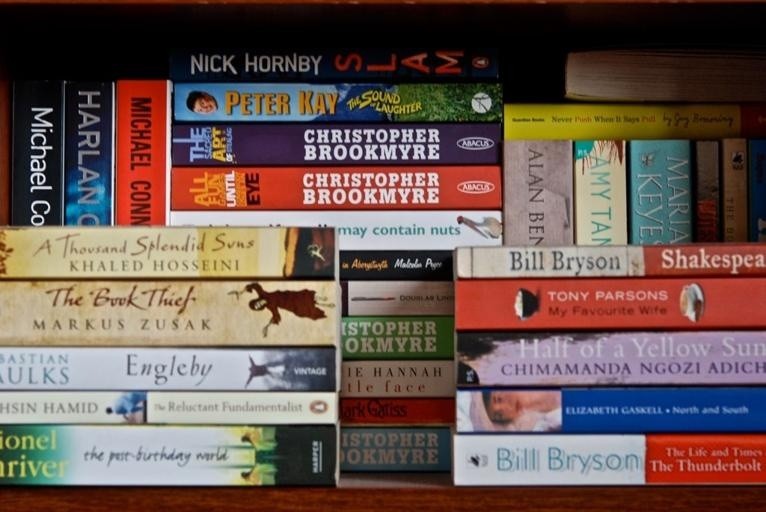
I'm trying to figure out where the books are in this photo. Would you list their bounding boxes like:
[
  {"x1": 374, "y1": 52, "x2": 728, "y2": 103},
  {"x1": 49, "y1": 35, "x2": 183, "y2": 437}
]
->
[{"x1": 2, "y1": 29, "x2": 766, "y2": 483}]
